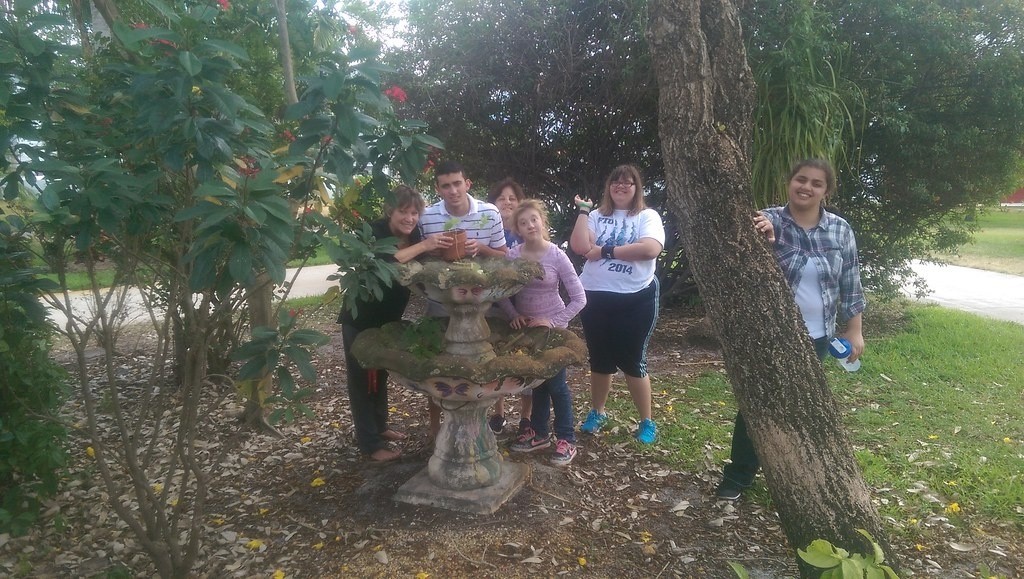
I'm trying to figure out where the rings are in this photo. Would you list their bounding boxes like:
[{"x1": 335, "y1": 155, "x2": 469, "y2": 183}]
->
[{"x1": 439, "y1": 240, "x2": 442, "y2": 244}]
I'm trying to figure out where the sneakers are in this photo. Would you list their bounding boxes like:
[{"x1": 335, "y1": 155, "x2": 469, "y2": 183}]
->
[
  {"x1": 718, "y1": 475, "x2": 745, "y2": 501},
  {"x1": 580, "y1": 408, "x2": 610, "y2": 435},
  {"x1": 487, "y1": 411, "x2": 507, "y2": 434},
  {"x1": 509, "y1": 426, "x2": 552, "y2": 452},
  {"x1": 636, "y1": 417, "x2": 658, "y2": 445},
  {"x1": 550, "y1": 439, "x2": 578, "y2": 467},
  {"x1": 517, "y1": 416, "x2": 532, "y2": 436}
]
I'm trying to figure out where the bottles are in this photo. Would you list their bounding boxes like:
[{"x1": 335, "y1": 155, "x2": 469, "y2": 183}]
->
[{"x1": 828, "y1": 338, "x2": 861, "y2": 372}]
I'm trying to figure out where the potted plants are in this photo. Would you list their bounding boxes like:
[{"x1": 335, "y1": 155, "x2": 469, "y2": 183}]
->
[{"x1": 442, "y1": 212, "x2": 492, "y2": 262}]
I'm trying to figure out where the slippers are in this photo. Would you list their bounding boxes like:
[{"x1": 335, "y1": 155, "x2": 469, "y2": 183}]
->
[
  {"x1": 382, "y1": 426, "x2": 409, "y2": 442},
  {"x1": 364, "y1": 437, "x2": 401, "y2": 461}
]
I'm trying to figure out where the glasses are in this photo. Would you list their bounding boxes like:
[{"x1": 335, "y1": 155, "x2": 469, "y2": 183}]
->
[{"x1": 611, "y1": 178, "x2": 636, "y2": 189}]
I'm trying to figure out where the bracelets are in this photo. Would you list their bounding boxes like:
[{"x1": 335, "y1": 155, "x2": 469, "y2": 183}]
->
[
  {"x1": 578, "y1": 202, "x2": 591, "y2": 209},
  {"x1": 578, "y1": 210, "x2": 589, "y2": 216}
]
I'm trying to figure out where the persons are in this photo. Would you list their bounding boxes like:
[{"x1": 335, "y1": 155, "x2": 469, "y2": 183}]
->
[
  {"x1": 336, "y1": 183, "x2": 454, "y2": 462},
  {"x1": 719, "y1": 158, "x2": 865, "y2": 503},
  {"x1": 417, "y1": 161, "x2": 508, "y2": 440},
  {"x1": 569, "y1": 164, "x2": 666, "y2": 444},
  {"x1": 488, "y1": 178, "x2": 587, "y2": 467}
]
